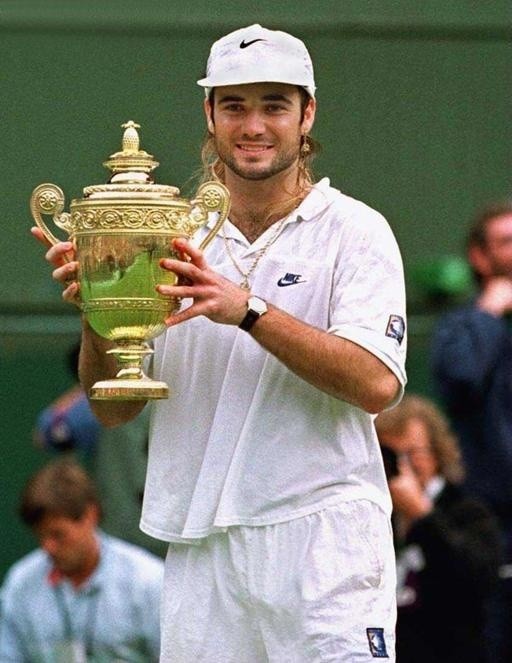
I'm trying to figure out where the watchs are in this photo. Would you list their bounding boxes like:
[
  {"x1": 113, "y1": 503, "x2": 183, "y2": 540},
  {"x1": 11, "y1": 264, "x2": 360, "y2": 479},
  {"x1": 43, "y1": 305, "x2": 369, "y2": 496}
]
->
[{"x1": 239, "y1": 295, "x2": 268, "y2": 332}]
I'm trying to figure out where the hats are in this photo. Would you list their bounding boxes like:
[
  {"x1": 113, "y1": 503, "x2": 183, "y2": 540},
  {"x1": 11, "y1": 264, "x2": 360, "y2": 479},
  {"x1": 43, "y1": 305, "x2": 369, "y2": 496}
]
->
[{"x1": 197, "y1": 24, "x2": 315, "y2": 99}]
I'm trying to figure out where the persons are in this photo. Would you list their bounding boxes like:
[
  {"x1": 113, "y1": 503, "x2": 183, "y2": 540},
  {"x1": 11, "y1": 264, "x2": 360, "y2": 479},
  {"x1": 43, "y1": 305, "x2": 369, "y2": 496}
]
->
[
  {"x1": 373, "y1": 204, "x2": 512, "y2": 663},
  {"x1": 1, "y1": 334, "x2": 161, "y2": 663},
  {"x1": 29, "y1": 24, "x2": 407, "y2": 663}
]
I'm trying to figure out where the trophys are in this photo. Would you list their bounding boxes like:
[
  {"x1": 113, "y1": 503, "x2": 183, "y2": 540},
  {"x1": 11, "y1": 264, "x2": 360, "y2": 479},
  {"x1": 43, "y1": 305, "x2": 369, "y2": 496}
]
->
[{"x1": 30, "y1": 120, "x2": 230, "y2": 399}]
